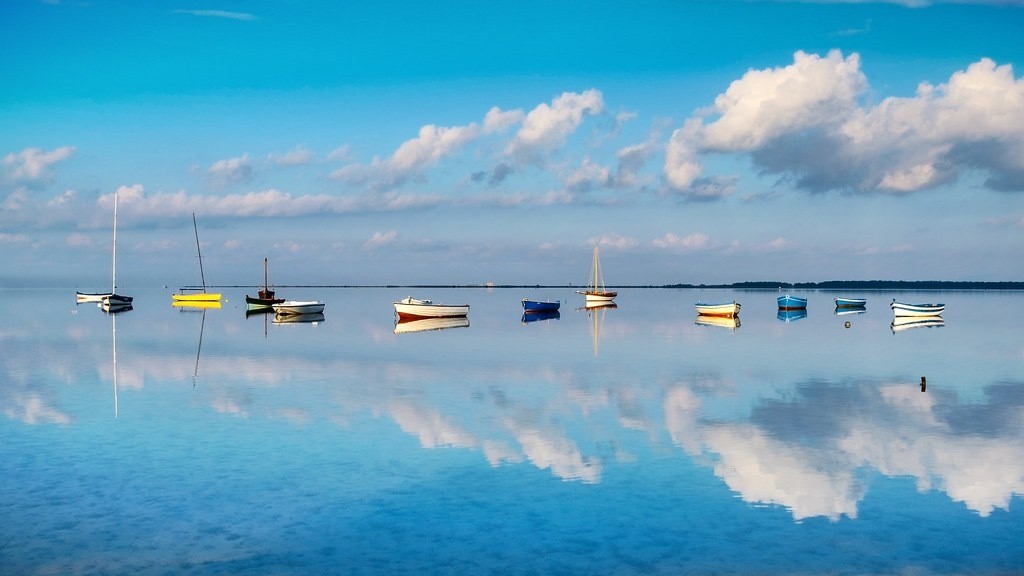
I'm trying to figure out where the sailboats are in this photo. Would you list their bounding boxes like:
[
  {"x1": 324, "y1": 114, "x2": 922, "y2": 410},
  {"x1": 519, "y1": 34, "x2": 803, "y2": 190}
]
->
[
  {"x1": 102, "y1": 305, "x2": 133, "y2": 419},
  {"x1": 585, "y1": 302, "x2": 618, "y2": 356},
  {"x1": 172, "y1": 301, "x2": 222, "y2": 387},
  {"x1": 246, "y1": 305, "x2": 274, "y2": 336},
  {"x1": 246, "y1": 257, "x2": 285, "y2": 305},
  {"x1": 171, "y1": 211, "x2": 221, "y2": 301},
  {"x1": 102, "y1": 191, "x2": 133, "y2": 304},
  {"x1": 584, "y1": 245, "x2": 618, "y2": 301}
]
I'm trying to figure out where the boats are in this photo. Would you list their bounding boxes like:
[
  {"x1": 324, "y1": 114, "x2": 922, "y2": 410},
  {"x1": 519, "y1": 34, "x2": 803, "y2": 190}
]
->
[
  {"x1": 834, "y1": 308, "x2": 866, "y2": 315},
  {"x1": 394, "y1": 297, "x2": 470, "y2": 318},
  {"x1": 272, "y1": 315, "x2": 324, "y2": 325},
  {"x1": 778, "y1": 295, "x2": 808, "y2": 310},
  {"x1": 889, "y1": 316, "x2": 945, "y2": 333},
  {"x1": 695, "y1": 314, "x2": 741, "y2": 333},
  {"x1": 776, "y1": 310, "x2": 807, "y2": 323},
  {"x1": 694, "y1": 300, "x2": 741, "y2": 316},
  {"x1": 522, "y1": 299, "x2": 560, "y2": 312},
  {"x1": 77, "y1": 298, "x2": 103, "y2": 305},
  {"x1": 271, "y1": 301, "x2": 326, "y2": 315},
  {"x1": 834, "y1": 296, "x2": 867, "y2": 308},
  {"x1": 76, "y1": 290, "x2": 106, "y2": 298},
  {"x1": 520, "y1": 311, "x2": 560, "y2": 324},
  {"x1": 393, "y1": 318, "x2": 470, "y2": 334},
  {"x1": 890, "y1": 299, "x2": 945, "y2": 317}
]
